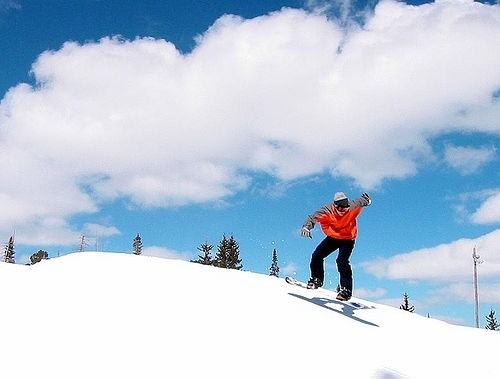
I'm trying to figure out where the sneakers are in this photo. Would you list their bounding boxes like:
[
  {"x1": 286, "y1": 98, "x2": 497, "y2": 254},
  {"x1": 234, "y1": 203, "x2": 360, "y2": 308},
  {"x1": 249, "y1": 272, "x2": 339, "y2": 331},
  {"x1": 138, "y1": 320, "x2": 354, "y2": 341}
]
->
[
  {"x1": 336, "y1": 289, "x2": 353, "y2": 301},
  {"x1": 307, "y1": 277, "x2": 324, "y2": 290}
]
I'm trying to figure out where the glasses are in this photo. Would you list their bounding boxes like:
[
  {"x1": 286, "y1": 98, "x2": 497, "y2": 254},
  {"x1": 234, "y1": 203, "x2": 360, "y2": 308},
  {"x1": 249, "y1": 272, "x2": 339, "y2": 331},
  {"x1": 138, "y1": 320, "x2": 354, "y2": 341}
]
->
[{"x1": 332, "y1": 198, "x2": 350, "y2": 208}]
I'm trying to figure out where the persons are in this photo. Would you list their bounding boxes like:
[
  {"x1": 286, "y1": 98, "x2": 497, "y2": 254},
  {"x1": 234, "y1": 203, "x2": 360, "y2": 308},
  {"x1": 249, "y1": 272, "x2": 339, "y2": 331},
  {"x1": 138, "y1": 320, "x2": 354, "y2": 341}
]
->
[{"x1": 300, "y1": 192, "x2": 372, "y2": 303}]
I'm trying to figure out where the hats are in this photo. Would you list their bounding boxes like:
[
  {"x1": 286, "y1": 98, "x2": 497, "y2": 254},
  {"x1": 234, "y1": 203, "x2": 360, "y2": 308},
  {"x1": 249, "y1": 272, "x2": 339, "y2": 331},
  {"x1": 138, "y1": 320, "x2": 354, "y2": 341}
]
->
[{"x1": 333, "y1": 191, "x2": 351, "y2": 208}]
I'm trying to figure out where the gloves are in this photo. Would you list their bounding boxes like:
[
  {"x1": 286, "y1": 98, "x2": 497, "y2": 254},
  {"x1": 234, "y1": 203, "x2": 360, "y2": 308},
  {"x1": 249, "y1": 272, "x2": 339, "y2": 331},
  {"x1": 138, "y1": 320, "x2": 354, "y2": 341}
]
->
[
  {"x1": 300, "y1": 227, "x2": 314, "y2": 240},
  {"x1": 361, "y1": 192, "x2": 372, "y2": 206}
]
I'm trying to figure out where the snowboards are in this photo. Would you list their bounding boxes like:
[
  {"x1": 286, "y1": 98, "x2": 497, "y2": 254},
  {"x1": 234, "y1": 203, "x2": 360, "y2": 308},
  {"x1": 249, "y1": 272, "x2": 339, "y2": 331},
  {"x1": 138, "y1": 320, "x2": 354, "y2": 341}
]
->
[{"x1": 286, "y1": 276, "x2": 375, "y2": 310}]
